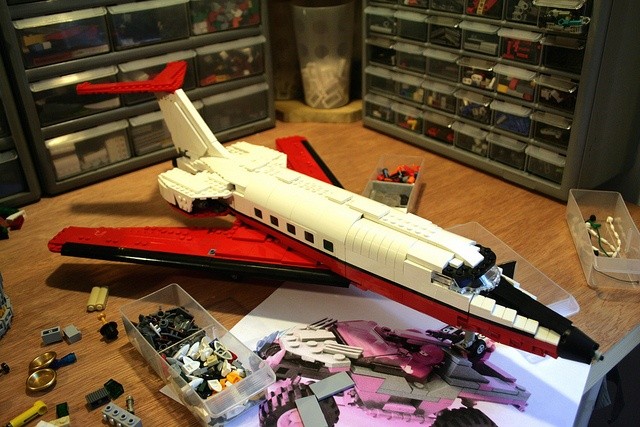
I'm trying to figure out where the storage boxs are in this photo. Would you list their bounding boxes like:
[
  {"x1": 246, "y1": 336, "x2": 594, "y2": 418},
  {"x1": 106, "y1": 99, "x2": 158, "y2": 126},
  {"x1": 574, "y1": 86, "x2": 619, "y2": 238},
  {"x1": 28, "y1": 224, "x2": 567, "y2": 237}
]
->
[
  {"x1": 503, "y1": 0, "x2": 541, "y2": 28},
  {"x1": 420, "y1": 79, "x2": 456, "y2": 119},
  {"x1": 524, "y1": 145, "x2": 566, "y2": 187},
  {"x1": 399, "y1": 0, "x2": 429, "y2": 10},
  {"x1": 388, "y1": 101, "x2": 423, "y2": 135},
  {"x1": 119, "y1": 51, "x2": 199, "y2": 106},
  {"x1": 394, "y1": 44, "x2": 426, "y2": 78},
  {"x1": 445, "y1": 220, "x2": 579, "y2": 321},
  {"x1": 455, "y1": 57, "x2": 494, "y2": 96},
  {"x1": 363, "y1": 38, "x2": 397, "y2": 68},
  {"x1": 421, "y1": 49, "x2": 458, "y2": 86},
  {"x1": 497, "y1": 27, "x2": 542, "y2": 67},
  {"x1": 458, "y1": 20, "x2": 499, "y2": 57},
  {"x1": 11, "y1": 8, "x2": 115, "y2": 70},
  {"x1": 489, "y1": 100, "x2": 533, "y2": 138},
  {"x1": 361, "y1": 152, "x2": 426, "y2": 213},
  {"x1": 566, "y1": 189, "x2": 640, "y2": 291},
  {"x1": 195, "y1": 35, "x2": 268, "y2": 87},
  {"x1": 364, "y1": 8, "x2": 395, "y2": 37},
  {"x1": 362, "y1": 66, "x2": 397, "y2": 100},
  {"x1": 419, "y1": 111, "x2": 456, "y2": 148},
  {"x1": 527, "y1": 110, "x2": 573, "y2": 152},
  {"x1": 202, "y1": 81, "x2": 272, "y2": 136},
  {"x1": 394, "y1": 73, "x2": 424, "y2": 108},
  {"x1": 451, "y1": 89, "x2": 493, "y2": 126},
  {"x1": 394, "y1": 10, "x2": 427, "y2": 43},
  {"x1": 362, "y1": 94, "x2": 393, "y2": 125},
  {"x1": 363, "y1": 0, "x2": 398, "y2": 7},
  {"x1": 0, "y1": 153, "x2": 38, "y2": 210},
  {"x1": 129, "y1": 99, "x2": 205, "y2": 157},
  {"x1": 538, "y1": 35, "x2": 586, "y2": 77},
  {"x1": 424, "y1": 15, "x2": 462, "y2": 49},
  {"x1": 533, "y1": 0, "x2": 593, "y2": 35},
  {"x1": 106, "y1": 0, "x2": 190, "y2": 50},
  {"x1": 29, "y1": 65, "x2": 121, "y2": 129},
  {"x1": 532, "y1": 74, "x2": 578, "y2": 118},
  {"x1": 43, "y1": 119, "x2": 133, "y2": 183},
  {"x1": 465, "y1": 0, "x2": 503, "y2": 20},
  {"x1": 449, "y1": 122, "x2": 490, "y2": 161},
  {"x1": 429, "y1": 1, "x2": 464, "y2": 16},
  {"x1": 484, "y1": 133, "x2": 527, "y2": 176},
  {"x1": 0, "y1": 84, "x2": 20, "y2": 152},
  {"x1": 118, "y1": 283, "x2": 277, "y2": 427},
  {"x1": 491, "y1": 64, "x2": 536, "y2": 106},
  {"x1": 189, "y1": 2, "x2": 262, "y2": 35}
]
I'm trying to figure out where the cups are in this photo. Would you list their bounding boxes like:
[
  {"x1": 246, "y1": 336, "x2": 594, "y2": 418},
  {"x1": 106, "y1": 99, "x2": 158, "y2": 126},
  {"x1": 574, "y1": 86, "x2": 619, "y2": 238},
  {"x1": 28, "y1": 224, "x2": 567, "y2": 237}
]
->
[{"x1": 291, "y1": 3, "x2": 353, "y2": 110}]
